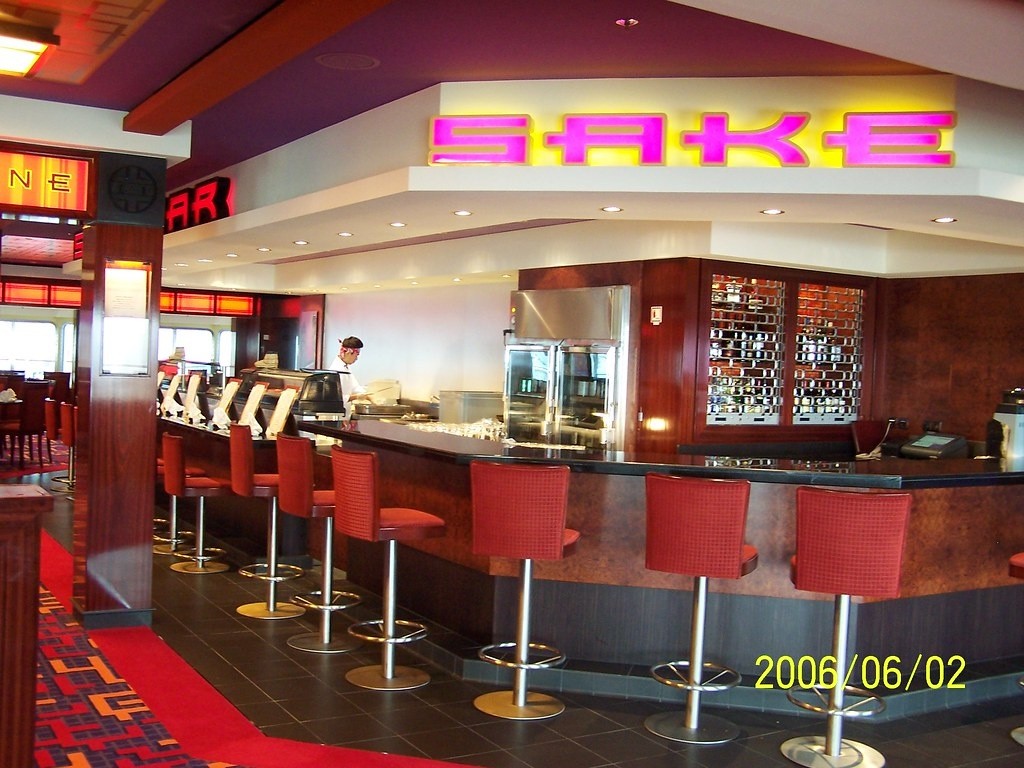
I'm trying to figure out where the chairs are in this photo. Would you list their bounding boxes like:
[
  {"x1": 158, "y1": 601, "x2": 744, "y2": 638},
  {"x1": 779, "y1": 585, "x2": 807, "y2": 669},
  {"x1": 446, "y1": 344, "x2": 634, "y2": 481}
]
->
[{"x1": 0, "y1": 370, "x2": 71, "y2": 470}]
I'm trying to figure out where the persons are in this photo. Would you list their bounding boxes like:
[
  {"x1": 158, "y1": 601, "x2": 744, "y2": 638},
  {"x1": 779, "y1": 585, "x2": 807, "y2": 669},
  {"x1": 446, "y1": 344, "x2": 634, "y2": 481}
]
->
[{"x1": 329, "y1": 336, "x2": 375, "y2": 420}]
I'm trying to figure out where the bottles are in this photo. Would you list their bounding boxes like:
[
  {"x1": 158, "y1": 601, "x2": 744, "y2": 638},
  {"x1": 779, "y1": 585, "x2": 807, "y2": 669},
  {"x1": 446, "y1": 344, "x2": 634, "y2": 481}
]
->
[{"x1": 707, "y1": 325, "x2": 846, "y2": 415}]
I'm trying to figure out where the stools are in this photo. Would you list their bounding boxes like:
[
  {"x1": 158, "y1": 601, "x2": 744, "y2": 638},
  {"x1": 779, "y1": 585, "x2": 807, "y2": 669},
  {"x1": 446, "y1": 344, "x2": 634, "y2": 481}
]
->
[
  {"x1": 779, "y1": 486, "x2": 912, "y2": 768},
  {"x1": 644, "y1": 472, "x2": 759, "y2": 745},
  {"x1": 153, "y1": 422, "x2": 447, "y2": 690},
  {"x1": 45, "y1": 397, "x2": 78, "y2": 502},
  {"x1": 1008, "y1": 551, "x2": 1024, "y2": 746},
  {"x1": 471, "y1": 459, "x2": 580, "y2": 720}
]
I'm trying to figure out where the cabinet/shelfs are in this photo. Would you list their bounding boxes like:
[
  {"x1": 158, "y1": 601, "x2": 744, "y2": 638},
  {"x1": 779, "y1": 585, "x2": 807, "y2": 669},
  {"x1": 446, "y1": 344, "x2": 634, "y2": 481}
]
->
[{"x1": 506, "y1": 346, "x2": 616, "y2": 460}]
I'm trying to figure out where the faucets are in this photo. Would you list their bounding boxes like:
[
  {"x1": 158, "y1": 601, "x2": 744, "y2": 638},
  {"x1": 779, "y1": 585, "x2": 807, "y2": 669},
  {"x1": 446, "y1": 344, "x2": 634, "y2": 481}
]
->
[{"x1": 429, "y1": 395, "x2": 440, "y2": 402}]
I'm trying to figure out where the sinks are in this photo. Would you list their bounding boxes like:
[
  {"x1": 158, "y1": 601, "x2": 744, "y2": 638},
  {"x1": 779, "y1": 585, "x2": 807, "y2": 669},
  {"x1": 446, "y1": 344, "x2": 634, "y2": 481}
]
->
[{"x1": 411, "y1": 414, "x2": 439, "y2": 420}]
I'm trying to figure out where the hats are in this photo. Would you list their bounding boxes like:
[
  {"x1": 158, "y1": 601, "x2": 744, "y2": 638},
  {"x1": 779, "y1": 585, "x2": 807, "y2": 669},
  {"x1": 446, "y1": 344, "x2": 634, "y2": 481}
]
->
[{"x1": 342, "y1": 336, "x2": 363, "y2": 349}]
